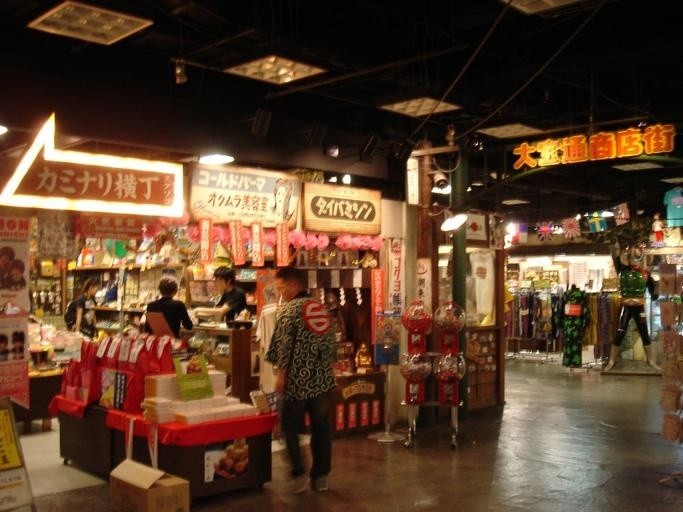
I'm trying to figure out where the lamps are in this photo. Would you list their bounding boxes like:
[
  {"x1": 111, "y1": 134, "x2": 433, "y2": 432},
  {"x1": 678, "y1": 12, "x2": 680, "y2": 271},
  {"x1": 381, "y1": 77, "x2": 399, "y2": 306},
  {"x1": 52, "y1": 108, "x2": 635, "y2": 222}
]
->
[{"x1": 175, "y1": 62, "x2": 188, "y2": 85}]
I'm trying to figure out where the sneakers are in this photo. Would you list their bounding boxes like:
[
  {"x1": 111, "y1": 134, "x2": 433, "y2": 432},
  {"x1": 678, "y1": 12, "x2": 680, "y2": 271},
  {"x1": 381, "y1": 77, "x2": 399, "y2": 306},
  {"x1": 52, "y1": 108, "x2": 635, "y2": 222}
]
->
[
  {"x1": 293, "y1": 473, "x2": 310, "y2": 494},
  {"x1": 311, "y1": 474, "x2": 328, "y2": 492}
]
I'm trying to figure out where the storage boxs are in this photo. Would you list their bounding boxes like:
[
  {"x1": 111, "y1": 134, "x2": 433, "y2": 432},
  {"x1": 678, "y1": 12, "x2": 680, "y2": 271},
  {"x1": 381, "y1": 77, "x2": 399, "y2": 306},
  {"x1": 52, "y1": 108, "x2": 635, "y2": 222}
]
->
[{"x1": 109, "y1": 459, "x2": 192, "y2": 512}]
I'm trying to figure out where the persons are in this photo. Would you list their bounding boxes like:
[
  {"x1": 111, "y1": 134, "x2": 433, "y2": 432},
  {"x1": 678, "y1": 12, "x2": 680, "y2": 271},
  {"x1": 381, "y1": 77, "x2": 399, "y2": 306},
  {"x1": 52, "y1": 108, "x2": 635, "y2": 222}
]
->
[
  {"x1": 264, "y1": 266, "x2": 338, "y2": 494},
  {"x1": 12, "y1": 331, "x2": 25, "y2": 360},
  {"x1": 0, "y1": 246, "x2": 15, "y2": 288},
  {"x1": 145, "y1": 278, "x2": 193, "y2": 338},
  {"x1": 0, "y1": 333, "x2": 10, "y2": 360},
  {"x1": 65, "y1": 275, "x2": 100, "y2": 334},
  {"x1": 213, "y1": 267, "x2": 246, "y2": 321},
  {"x1": 651, "y1": 215, "x2": 664, "y2": 242},
  {"x1": 8, "y1": 259, "x2": 26, "y2": 292},
  {"x1": 601, "y1": 242, "x2": 664, "y2": 371},
  {"x1": 554, "y1": 284, "x2": 591, "y2": 367}
]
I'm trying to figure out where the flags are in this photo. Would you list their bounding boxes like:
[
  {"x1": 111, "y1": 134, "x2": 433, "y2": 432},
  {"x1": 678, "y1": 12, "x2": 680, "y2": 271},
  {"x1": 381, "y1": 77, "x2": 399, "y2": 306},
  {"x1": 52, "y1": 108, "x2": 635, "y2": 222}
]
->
[
  {"x1": 561, "y1": 217, "x2": 582, "y2": 239},
  {"x1": 511, "y1": 223, "x2": 529, "y2": 244},
  {"x1": 536, "y1": 221, "x2": 553, "y2": 242},
  {"x1": 612, "y1": 203, "x2": 630, "y2": 226},
  {"x1": 586, "y1": 209, "x2": 608, "y2": 232}
]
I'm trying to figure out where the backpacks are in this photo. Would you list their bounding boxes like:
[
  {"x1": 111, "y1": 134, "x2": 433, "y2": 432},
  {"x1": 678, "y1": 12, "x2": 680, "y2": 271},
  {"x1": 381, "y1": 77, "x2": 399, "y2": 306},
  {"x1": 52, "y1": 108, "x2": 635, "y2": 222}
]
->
[{"x1": 65, "y1": 295, "x2": 97, "y2": 328}]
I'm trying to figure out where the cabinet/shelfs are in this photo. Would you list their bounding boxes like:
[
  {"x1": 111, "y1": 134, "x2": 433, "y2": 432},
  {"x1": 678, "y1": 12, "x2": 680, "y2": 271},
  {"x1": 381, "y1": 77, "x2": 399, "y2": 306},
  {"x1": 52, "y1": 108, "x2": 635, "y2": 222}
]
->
[{"x1": 70, "y1": 255, "x2": 277, "y2": 350}]
